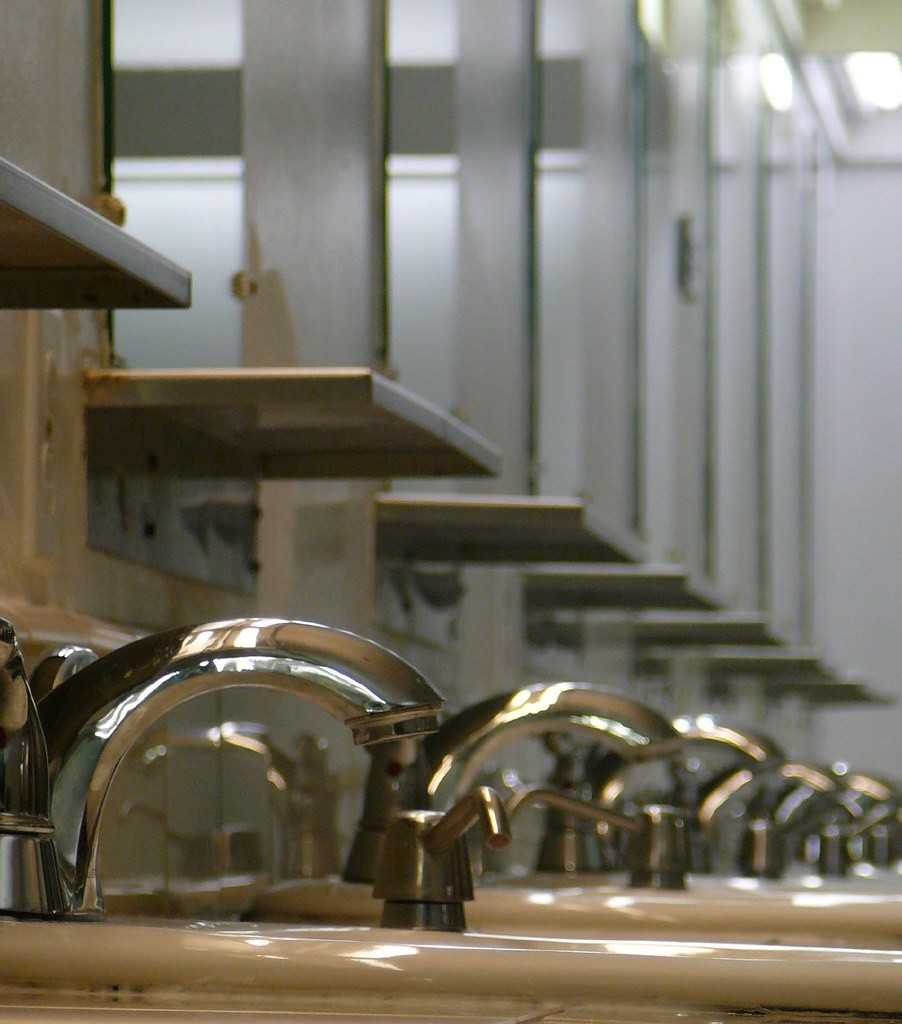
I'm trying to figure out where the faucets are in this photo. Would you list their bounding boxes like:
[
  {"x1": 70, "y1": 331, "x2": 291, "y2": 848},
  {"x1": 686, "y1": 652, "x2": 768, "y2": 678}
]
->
[
  {"x1": 383, "y1": 681, "x2": 898, "y2": 888},
  {"x1": 32, "y1": 617, "x2": 446, "y2": 922}
]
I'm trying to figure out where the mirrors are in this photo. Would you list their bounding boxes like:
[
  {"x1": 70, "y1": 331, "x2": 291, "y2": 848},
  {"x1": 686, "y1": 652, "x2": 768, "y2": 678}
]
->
[{"x1": 83, "y1": 0, "x2": 823, "y2": 709}]
